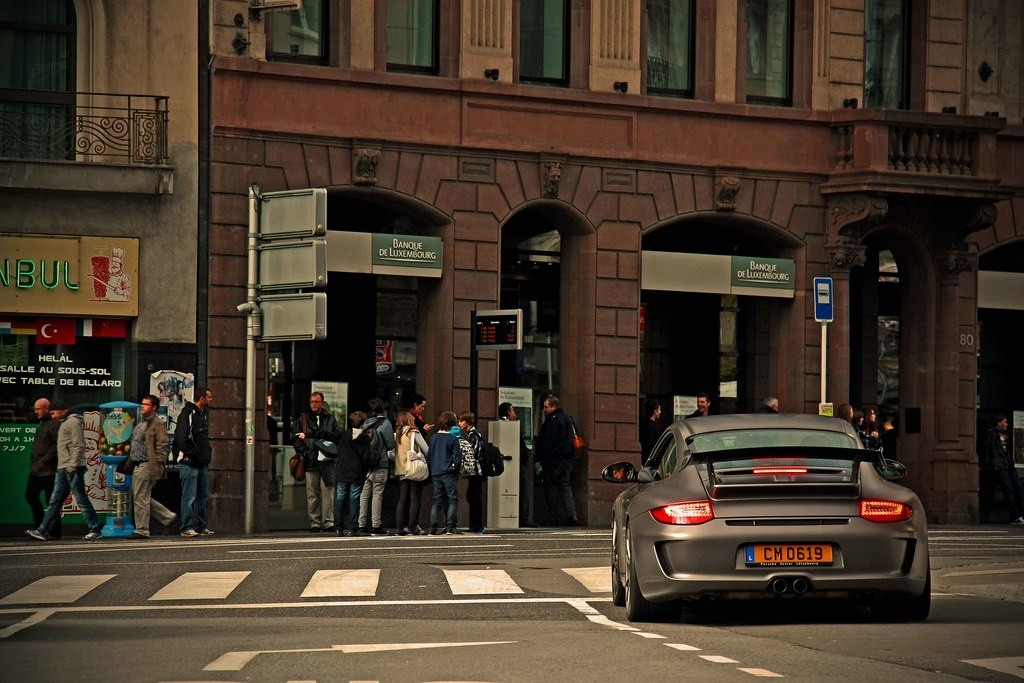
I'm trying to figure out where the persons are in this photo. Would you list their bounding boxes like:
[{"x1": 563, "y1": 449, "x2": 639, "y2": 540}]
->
[
  {"x1": 604, "y1": 464, "x2": 627, "y2": 481},
  {"x1": 837, "y1": 404, "x2": 897, "y2": 460},
  {"x1": 175, "y1": 387, "x2": 215, "y2": 537},
  {"x1": 27, "y1": 400, "x2": 102, "y2": 541},
  {"x1": 534, "y1": 394, "x2": 581, "y2": 526},
  {"x1": 984, "y1": 415, "x2": 1024, "y2": 525},
  {"x1": 498, "y1": 402, "x2": 538, "y2": 527},
  {"x1": 684, "y1": 393, "x2": 713, "y2": 420},
  {"x1": 639, "y1": 399, "x2": 662, "y2": 468},
  {"x1": 26, "y1": 398, "x2": 62, "y2": 540},
  {"x1": 127, "y1": 395, "x2": 179, "y2": 539},
  {"x1": 267, "y1": 391, "x2": 488, "y2": 536},
  {"x1": 756, "y1": 396, "x2": 780, "y2": 414}
]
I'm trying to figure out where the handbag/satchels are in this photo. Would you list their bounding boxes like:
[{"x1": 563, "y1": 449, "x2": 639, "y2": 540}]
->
[
  {"x1": 387, "y1": 432, "x2": 397, "y2": 462},
  {"x1": 571, "y1": 422, "x2": 586, "y2": 459},
  {"x1": 405, "y1": 430, "x2": 429, "y2": 481},
  {"x1": 290, "y1": 454, "x2": 304, "y2": 481}
]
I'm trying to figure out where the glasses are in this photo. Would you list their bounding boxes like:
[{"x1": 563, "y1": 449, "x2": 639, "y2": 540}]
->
[{"x1": 309, "y1": 400, "x2": 322, "y2": 403}]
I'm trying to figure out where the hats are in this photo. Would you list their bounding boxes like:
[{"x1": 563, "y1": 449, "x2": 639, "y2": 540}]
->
[
  {"x1": 645, "y1": 400, "x2": 658, "y2": 418},
  {"x1": 450, "y1": 426, "x2": 461, "y2": 438},
  {"x1": 49, "y1": 400, "x2": 65, "y2": 410}
]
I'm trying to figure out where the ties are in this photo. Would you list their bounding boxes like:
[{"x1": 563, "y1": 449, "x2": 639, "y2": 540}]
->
[{"x1": 316, "y1": 416, "x2": 320, "y2": 429}]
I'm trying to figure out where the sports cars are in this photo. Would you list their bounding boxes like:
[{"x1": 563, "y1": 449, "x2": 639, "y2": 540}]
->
[{"x1": 600, "y1": 413, "x2": 931, "y2": 622}]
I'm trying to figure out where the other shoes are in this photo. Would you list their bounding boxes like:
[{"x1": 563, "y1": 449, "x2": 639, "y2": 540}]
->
[
  {"x1": 127, "y1": 534, "x2": 148, "y2": 539},
  {"x1": 46, "y1": 529, "x2": 62, "y2": 540},
  {"x1": 163, "y1": 516, "x2": 180, "y2": 534},
  {"x1": 310, "y1": 524, "x2": 483, "y2": 536}
]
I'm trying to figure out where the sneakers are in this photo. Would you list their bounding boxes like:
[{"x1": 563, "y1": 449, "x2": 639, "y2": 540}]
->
[
  {"x1": 1010, "y1": 517, "x2": 1024, "y2": 525},
  {"x1": 181, "y1": 528, "x2": 198, "y2": 537},
  {"x1": 85, "y1": 531, "x2": 103, "y2": 540},
  {"x1": 24, "y1": 529, "x2": 47, "y2": 541},
  {"x1": 197, "y1": 528, "x2": 214, "y2": 536}
]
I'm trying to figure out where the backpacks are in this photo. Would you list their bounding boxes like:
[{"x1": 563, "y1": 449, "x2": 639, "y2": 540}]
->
[
  {"x1": 365, "y1": 419, "x2": 384, "y2": 468},
  {"x1": 468, "y1": 431, "x2": 504, "y2": 477},
  {"x1": 458, "y1": 438, "x2": 476, "y2": 479}
]
